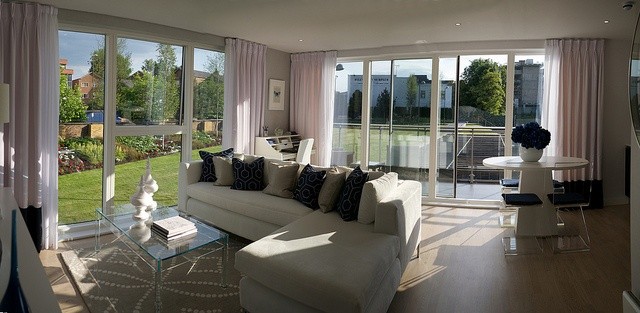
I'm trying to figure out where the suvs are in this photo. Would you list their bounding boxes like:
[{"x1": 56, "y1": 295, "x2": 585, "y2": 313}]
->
[{"x1": 87, "y1": 110, "x2": 121, "y2": 124}]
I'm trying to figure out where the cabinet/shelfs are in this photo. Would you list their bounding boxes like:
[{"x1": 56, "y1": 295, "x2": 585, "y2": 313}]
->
[{"x1": 255, "y1": 133, "x2": 316, "y2": 161}]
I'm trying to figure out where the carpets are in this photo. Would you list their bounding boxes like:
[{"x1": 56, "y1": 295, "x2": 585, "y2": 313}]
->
[{"x1": 60, "y1": 214, "x2": 253, "y2": 312}]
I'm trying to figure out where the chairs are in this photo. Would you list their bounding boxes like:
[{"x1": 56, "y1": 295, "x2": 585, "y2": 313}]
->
[{"x1": 295, "y1": 137, "x2": 315, "y2": 162}]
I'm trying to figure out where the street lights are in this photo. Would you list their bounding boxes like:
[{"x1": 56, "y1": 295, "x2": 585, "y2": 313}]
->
[
  {"x1": 417, "y1": 81, "x2": 426, "y2": 124},
  {"x1": 443, "y1": 86, "x2": 449, "y2": 124}
]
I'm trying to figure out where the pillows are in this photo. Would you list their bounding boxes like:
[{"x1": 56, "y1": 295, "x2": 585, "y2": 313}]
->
[
  {"x1": 293, "y1": 163, "x2": 326, "y2": 210},
  {"x1": 318, "y1": 166, "x2": 347, "y2": 214},
  {"x1": 198, "y1": 147, "x2": 235, "y2": 183},
  {"x1": 357, "y1": 171, "x2": 399, "y2": 225},
  {"x1": 337, "y1": 165, "x2": 370, "y2": 222},
  {"x1": 212, "y1": 153, "x2": 244, "y2": 186},
  {"x1": 263, "y1": 159, "x2": 299, "y2": 199},
  {"x1": 229, "y1": 155, "x2": 265, "y2": 191}
]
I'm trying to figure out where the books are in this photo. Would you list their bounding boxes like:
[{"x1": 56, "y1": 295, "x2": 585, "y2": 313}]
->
[{"x1": 151, "y1": 215, "x2": 197, "y2": 241}]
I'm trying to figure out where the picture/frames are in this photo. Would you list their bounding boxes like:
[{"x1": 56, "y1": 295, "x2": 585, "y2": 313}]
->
[{"x1": 268, "y1": 78, "x2": 286, "y2": 111}]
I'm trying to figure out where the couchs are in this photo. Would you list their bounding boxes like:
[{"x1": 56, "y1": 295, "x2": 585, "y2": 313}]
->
[{"x1": 178, "y1": 153, "x2": 422, "y2": 312}]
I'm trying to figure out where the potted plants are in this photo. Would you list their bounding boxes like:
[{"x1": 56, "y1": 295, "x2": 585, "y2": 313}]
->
[{"x1": 512, "y1": 121, "x2": 551, "y2": 161}]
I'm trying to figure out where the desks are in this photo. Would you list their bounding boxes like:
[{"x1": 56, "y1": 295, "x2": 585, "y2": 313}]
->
[{"x1": 482, "y1": 153, "x2": 596, "y2": 257}]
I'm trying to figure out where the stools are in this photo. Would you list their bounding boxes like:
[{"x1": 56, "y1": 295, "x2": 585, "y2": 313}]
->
[
  {"x1": 499, "y1": 176, "x2": 520, "y2": 228},
  {"x1": 548, "y1": 191, "x2": 594, "y2": 254},
  {"x1": 500, "y1": 192, "x2": 543, "y2": 257},
  {"x1": 548, "y1": 181, "x2": 566, "y2": 227}
]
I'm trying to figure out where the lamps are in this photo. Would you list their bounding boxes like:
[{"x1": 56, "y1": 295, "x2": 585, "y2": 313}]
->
[{"x1": 0, "y1": 81, "x2": 10, "y2": 124}]
